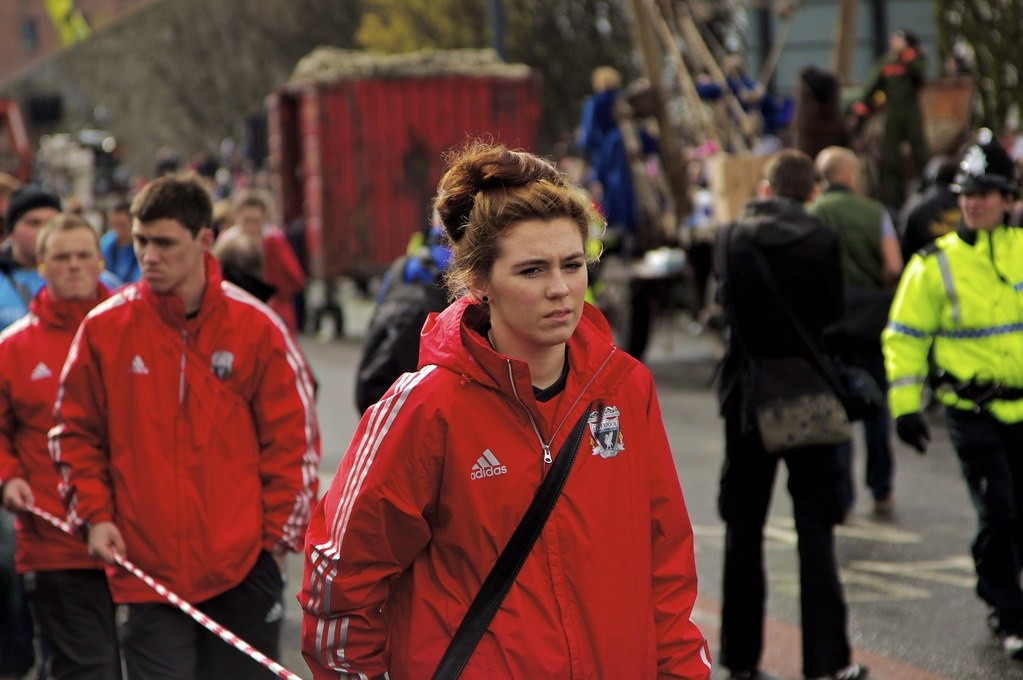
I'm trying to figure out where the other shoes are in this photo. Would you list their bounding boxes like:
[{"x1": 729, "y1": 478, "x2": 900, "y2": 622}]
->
[
  {"x1": 804, "y1": 663, "x2": 866, "y2": 680},
  {"x1": 730, "y1": 670, "x2": 774, "y2": 680},
  {"x1": 1003, "y1": 634, "x2": 1023, "y2": 656},
  {"x1": 877, "y1": 493, "x2": 894, "y2": 512}
]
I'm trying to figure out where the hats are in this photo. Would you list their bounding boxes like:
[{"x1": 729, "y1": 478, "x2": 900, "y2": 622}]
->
[{"x1": 946, "y1": 128, "x2": 1014, "y2": 195}]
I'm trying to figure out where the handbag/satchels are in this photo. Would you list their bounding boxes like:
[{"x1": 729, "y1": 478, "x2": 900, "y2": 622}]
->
[{"x1": 754, "y1": 394, "x2": 853, "y2": 451}]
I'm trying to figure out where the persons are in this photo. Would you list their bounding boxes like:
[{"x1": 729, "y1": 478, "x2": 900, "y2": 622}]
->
[
  {"x1": 574, "y1": 26, "x2": 1023, "y2": 524},
  {"x1": 352, "y1": 203, "x2": 460, "y2": 421},
  {"x1": 0, "y1": 157, "x2": 306, "y2": 679},
  {"x1": 297, "y1": 145, "x2": 712, "y2": 680},
  {"x1": 706, "y1": 150, "x2": 872, "y2": 680},
  {"x1": 880, "y1": 139, "x2": 1023, "y2": 658},
  {"x1": 48, "y1": 175, "x2": 319, "y2": 680}
]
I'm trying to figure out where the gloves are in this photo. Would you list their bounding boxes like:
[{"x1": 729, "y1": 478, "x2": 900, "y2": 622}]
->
[{"x1": 897, "y1": 412, "x2": 932, "y2": 454}]
